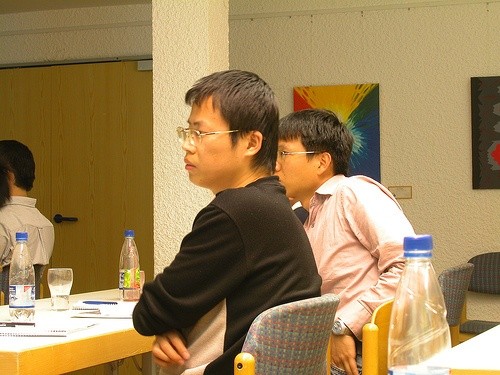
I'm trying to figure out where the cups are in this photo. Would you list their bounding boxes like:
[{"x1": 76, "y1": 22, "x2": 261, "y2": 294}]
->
[
  {"x1": 47, "y1": 268, "x2": 73, "y2": 311},
  {"x1": 123, "y1": 271, "x2": 146, "y2": 300}
]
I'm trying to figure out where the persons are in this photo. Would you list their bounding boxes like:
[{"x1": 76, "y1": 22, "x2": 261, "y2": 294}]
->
[
  {"x1": 0, "y1": 140, "x2": 54, "y2": 306},
  {"x1": 274, "y1": 108, "x2": 432, "y2": 375},
  {"x1": 133, "y1": 71, "x2": 322, "y2": 375}
]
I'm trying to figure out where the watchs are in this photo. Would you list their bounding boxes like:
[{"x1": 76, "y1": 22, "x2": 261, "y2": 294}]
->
[{"x1": 331, "y1": 319, "x2": 353, "y2": 336}]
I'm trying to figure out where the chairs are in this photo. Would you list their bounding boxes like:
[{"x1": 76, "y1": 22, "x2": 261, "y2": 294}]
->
[{"x1": 233, "y1": 252, "x2": 500, "y2": 375}]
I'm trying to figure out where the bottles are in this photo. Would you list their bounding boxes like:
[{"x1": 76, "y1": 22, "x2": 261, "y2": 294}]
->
[
  {"x1": 8, "y1": 232, "x2": 36, "y2": 326},
  {"x1": 119, "y1": 229, "x2": 141, "y2": 302},
  {"x1": 387, "y1": 233, "x2": 453, "y2": 375}
]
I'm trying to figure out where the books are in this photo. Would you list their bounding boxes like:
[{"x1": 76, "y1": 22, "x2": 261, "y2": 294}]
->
[
  {"x1": 0, "y1": 319, "x2": 98, "y2": 337},
  {"x1": 70, "y1": 299, "x2": 137, "y2": 319}
]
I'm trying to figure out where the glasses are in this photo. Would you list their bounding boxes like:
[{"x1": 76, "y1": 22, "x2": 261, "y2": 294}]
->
[
  {"x1": 177, "y1": 127, "x2": 266, "y2": 145},
  {"x1": 277, "y1": 151, "x2": 322, "y2": 163}
]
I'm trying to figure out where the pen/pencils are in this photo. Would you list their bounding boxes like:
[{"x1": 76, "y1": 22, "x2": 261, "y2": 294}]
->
[{"x1": 83, "y1": 301, "x2": 118, "y2": 305}]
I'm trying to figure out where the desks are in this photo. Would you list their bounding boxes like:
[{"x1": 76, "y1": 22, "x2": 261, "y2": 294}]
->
[
  {"x1": 0, "y1": 287, "x2": 157, "y2": 375},
  {"x1": 387, "y1": 324, "x2": 500, "y2": 375}
]
